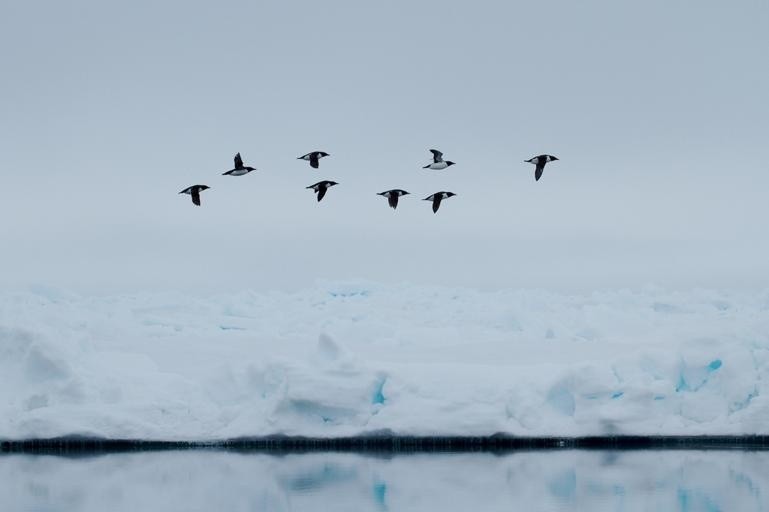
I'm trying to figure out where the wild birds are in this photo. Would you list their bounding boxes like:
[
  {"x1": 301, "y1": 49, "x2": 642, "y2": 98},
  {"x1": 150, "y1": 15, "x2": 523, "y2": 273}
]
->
[
  {"x1": 177, "y1": 185, "x2": 211, "y2": 206},
  {"x1": 221, "y1": 152, "x2": 257, "y2": 176},
  {"x1": 376, "y1": 189, "x2": 410, "y2": 210},
  {"x1": 523, "y1": 154, "x2": 559, "y2": 182},
  {"x1": 297, "y1": 151, "x2": 330, "y2": 169},
  {"x1": 422, "y1": 191, "x2": 456, "y2": 214},
  {"x1": 306, "y1": 180, "x2": 339, "y2": 202},
  {"x1": 421, "y1": 148, "x2": 456, "y2": 170}
]
